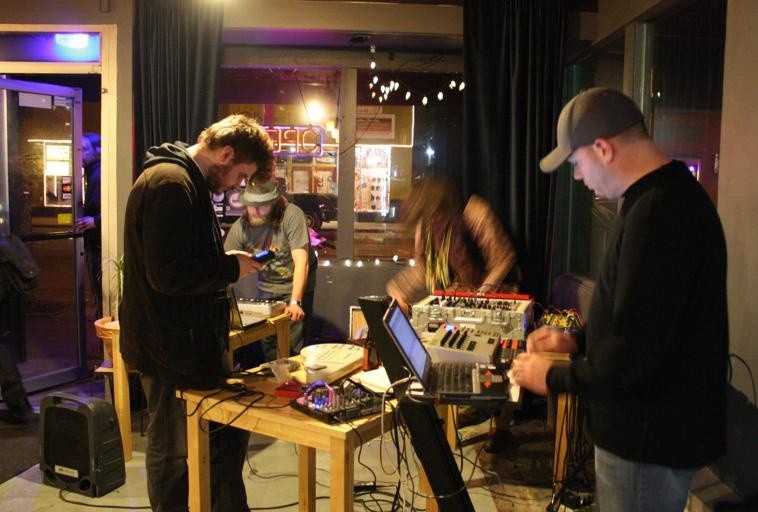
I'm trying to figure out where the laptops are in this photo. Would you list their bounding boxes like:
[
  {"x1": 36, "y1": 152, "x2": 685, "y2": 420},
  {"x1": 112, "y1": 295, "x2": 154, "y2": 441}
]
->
[
  {"x1": 227, "y1": 285, "x2": 267, "y2": 330},
  {"x1": 382, "y1": 296, "x2": 511, "y2": 401}
]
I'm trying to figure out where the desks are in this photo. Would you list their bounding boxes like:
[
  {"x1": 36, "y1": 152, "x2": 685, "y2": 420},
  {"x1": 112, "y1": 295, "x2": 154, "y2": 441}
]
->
[
  {"x1": 175, "y1": 355, "x2": 448, "y2": 512},
  {"x1": 448, "y1": 351, "x2": 574, "y2": 512},
  {"x1": 101, "y1": 299, "x2": 290, "y2": 462},
  {"x1": 94, "y1": 361, "x2": 145, "y2": 438}
]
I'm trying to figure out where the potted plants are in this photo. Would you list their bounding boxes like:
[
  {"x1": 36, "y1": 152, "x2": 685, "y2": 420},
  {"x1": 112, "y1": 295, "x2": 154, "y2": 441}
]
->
[{"x1": 94, "y1": 253, "x2": 126, "y2": 366}]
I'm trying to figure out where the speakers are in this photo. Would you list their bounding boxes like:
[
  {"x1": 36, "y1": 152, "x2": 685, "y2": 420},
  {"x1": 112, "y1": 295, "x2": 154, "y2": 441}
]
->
[{"x1": 38, "y1": 393, "x2": 126, "y2": 498}]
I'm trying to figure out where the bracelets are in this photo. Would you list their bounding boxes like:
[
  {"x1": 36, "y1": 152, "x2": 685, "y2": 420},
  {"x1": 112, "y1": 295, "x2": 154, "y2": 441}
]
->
[{"x1": 290, "y1": 300, "x2": 302, "y2": 306}]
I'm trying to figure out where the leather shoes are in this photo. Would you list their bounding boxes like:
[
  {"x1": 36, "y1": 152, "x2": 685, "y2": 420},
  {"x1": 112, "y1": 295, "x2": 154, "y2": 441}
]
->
[{"x1": 6, "y1": 412, "x2": 41, "y2": 423}]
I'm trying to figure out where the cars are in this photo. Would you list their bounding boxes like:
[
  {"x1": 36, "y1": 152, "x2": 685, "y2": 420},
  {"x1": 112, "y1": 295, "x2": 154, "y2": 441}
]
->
[{"x1": 276, "y1": 175, "x2": 337, "y2": 231}]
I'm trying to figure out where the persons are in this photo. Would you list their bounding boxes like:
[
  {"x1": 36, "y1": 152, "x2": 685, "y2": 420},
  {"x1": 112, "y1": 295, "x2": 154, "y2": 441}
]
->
[
  {"x1": 73, "y1": 133, "x2": 102, "y2": 319},
  {"x1": 0, "y1": 234, "x2": 34, "y2": 425},
  {"x1": 118, "y1": 114, "x2": 274, "y2": 512},
  {"x1": 511, "y1": 86, "x2": 728, "y2": 512},
  {"x1": 222, "y1": 173, "x2": 317, "y2": 362},
  {"x1": 386, "y1": 172, "x2": 523, "y2": 453}
]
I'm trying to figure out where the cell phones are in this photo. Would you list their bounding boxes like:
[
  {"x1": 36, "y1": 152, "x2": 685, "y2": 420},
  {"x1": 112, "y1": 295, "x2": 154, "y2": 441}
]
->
[{"x1": 248, "y1": 249, "x2": 275, "y2": 262}]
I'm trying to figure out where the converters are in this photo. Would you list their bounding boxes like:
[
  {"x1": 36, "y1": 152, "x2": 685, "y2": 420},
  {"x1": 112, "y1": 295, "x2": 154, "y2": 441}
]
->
[{"x1": 354, "y1": 481, "x2": 376, "y2": 492}]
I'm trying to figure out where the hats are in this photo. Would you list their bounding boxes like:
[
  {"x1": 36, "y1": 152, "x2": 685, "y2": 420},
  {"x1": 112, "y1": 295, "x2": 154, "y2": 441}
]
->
[
  {"x1": 238, "y1": 178, "x2": 286, "y2": 207},
  {"x1": 539, "y1": 86, "x2": 644, "y2": 174}
]
